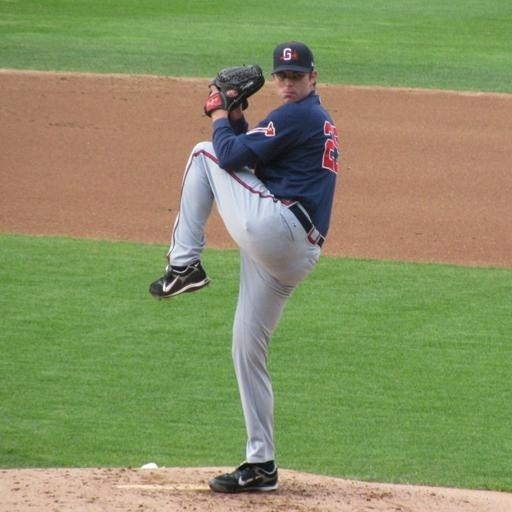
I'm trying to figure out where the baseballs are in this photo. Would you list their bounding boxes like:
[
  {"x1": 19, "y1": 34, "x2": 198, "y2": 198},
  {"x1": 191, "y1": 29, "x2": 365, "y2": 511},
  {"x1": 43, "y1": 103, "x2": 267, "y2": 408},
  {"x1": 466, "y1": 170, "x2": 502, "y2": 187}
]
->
[{"x1": 142, "y1": 462, "x2": 158, "y2": 469}]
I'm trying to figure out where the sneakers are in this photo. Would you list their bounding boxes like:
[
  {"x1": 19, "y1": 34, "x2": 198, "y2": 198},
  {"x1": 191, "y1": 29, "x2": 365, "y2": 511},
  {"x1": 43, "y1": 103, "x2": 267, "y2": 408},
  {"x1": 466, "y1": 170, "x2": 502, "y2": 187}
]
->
[
  {"x1": 209, "y1": 462, "x2": 279, "y2": 493},
  {"x1": 149, "y1": 261, "x2": 211, "y2": 299}
]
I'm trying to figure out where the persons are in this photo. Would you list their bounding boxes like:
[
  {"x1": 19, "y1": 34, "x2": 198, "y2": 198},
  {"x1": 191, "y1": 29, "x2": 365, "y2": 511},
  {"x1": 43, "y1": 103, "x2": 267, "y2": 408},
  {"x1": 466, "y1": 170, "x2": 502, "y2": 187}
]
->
[{"x1": 148, "y1": 40, "x2": 340, "y2": 493}]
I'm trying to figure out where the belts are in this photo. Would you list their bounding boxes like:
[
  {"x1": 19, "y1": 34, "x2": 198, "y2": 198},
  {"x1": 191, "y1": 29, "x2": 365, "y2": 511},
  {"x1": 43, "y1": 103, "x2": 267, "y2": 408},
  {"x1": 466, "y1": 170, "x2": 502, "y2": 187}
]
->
[{"x1": 290, "y1": 203, "x2": 326, "y2": 247}]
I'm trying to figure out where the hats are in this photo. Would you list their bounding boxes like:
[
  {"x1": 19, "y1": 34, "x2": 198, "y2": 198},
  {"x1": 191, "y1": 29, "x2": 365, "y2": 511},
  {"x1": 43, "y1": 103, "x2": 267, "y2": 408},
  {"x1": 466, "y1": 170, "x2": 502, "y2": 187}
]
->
[{"x1": 270, "y1": 40, "x2": 314, "y2": 73}]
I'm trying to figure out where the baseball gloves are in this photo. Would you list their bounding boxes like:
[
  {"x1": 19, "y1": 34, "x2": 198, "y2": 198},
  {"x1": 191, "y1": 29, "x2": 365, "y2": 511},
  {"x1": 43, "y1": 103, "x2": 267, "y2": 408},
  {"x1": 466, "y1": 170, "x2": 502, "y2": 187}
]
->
[{"x1": 204, "y1": 64, "x2": 264, "y2": 118}]
